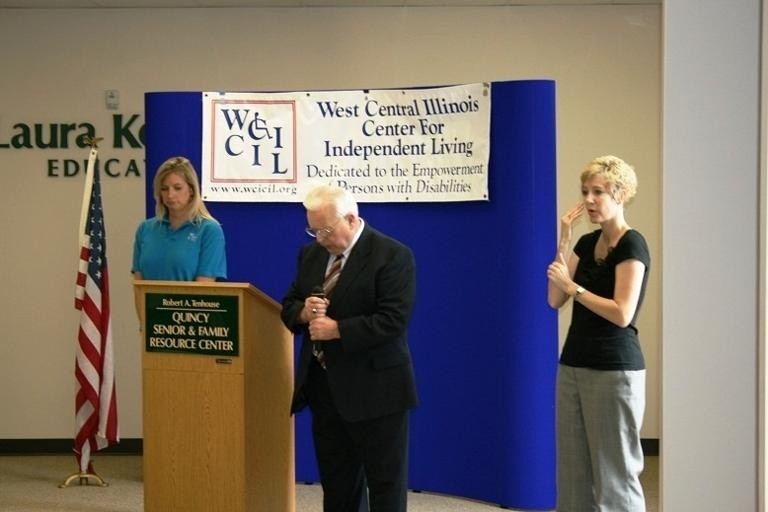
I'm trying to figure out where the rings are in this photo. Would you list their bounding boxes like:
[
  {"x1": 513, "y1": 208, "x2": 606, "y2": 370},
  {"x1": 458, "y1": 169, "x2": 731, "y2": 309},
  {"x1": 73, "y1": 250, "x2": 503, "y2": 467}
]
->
[{"x1": 312, "y1": 308, "x2": 317, "y2": 314}]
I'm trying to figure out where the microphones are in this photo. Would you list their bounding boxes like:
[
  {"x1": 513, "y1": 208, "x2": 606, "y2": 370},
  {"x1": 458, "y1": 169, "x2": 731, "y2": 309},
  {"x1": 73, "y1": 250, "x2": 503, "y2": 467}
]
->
[{"x1": 310, "y1": 284, "x2": 326, "y2": 357}]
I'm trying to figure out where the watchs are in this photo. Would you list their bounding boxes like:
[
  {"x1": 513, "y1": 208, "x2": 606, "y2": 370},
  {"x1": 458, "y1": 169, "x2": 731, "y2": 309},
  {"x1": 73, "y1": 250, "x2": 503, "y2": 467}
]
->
[{"x1": 574, "y1": 284, "x2": 586, "y2": 301}]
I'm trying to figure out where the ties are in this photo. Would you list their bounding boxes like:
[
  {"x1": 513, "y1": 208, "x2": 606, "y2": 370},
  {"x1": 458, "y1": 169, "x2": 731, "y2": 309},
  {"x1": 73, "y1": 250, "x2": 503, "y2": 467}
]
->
[{"x1": 310, "y1": 253, "x2": 345, "y2": 371}]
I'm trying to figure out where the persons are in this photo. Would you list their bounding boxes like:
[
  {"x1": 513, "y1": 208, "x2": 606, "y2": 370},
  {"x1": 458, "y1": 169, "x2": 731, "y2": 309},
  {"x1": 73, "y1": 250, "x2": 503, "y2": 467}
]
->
[
  {"x1": 280, "y1": 185, "x2": 419, "y2": 511},
  {"x1": 130, "y1": 156, "x2": 231, "y2": 336},
  {"x1": 544, "y1": 154, "x2": 649, "y2": 512}
]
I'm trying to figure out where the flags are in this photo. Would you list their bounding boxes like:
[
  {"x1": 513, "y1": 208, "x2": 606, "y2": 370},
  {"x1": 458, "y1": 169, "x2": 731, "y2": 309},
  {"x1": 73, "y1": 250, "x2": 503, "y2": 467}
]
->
[{"x1": 72, "y1": 149, "x2": 123, "y2": 474}]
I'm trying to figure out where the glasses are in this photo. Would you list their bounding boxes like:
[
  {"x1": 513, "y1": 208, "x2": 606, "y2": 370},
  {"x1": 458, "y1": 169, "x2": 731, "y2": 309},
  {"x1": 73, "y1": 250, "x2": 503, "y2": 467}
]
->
[{"x1": 304, "y1": 217, "x2": 345, "y2": 238}]
